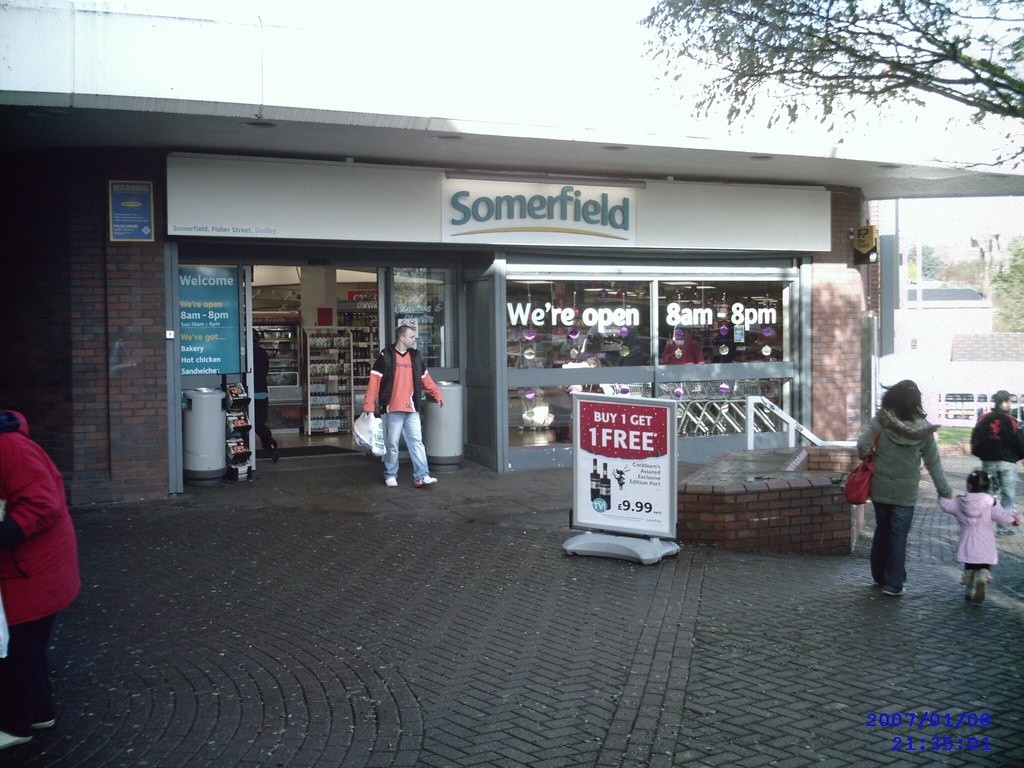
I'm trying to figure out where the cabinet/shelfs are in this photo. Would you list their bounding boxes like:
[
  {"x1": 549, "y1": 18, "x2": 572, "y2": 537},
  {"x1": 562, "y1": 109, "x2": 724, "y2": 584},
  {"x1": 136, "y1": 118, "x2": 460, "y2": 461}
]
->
[
  {"x1": 300, "y1": 325, "x2": 441, "y2": 436},
  {"x1": 506, "y1": 322, "x2": 572, "y2": 428}
]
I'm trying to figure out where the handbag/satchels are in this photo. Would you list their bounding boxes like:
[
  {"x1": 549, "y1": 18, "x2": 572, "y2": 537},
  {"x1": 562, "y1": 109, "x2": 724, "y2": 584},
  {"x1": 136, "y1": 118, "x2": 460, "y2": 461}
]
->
[
  {"x1": 843, "y1": 426, "x2": 880, "y2": 506},
  {"x1": 351, "y1": 411, "x2": 387, "y2": 457}
]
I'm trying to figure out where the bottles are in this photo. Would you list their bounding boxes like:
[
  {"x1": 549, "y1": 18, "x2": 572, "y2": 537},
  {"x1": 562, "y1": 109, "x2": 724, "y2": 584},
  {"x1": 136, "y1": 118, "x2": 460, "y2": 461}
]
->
[
  {"x1": 590, "y1": 458, "x2": 600, "y2": 501},
  {"x1": 310, "y1": 312, "x2": 379, "y2": 429},
  {"x1": 600, "y1": 463, "x2": 611, "y2": 510}
]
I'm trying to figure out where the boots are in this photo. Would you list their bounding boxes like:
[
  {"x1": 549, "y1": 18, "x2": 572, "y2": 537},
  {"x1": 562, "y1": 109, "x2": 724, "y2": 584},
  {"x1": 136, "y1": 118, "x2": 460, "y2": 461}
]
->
[
  {"x1": 960, "y1": 570, "x2": 975, "y2": 601},
  {"x1": 972, "y1": 568, "x2": 992, "y2": 602}
]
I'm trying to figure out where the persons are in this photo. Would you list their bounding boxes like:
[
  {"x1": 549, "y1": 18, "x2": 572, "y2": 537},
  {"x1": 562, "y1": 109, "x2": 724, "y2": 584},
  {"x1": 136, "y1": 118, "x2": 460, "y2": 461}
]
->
[
  {"x1": 547, "y1": 319, "x2": 740, "y2": 444},
  {"x1": 858, "y1": 381, "x2": 951, "y2": 596},
  {"x1": 364, "y1": 324, "x2": 445, "y2": 486},
  {"x1": 243, "y1": 328, "x2": 279, "y2": 463},
  {"x1": 971, "y1": 390, "x2": 1024, "y2": 534},
  {"x1": 0, "y1": 409, "x2": 81, "y2": 749},
  {"x1": 938, "y1": 470, "x2": 1020, "y2": 602}
]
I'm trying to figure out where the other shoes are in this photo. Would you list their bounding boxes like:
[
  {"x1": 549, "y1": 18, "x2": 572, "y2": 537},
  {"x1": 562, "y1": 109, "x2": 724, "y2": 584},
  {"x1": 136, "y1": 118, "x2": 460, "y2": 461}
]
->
[
  {"x1": 414, "y1": 475, "x2": 438, "y2": 487},
  {"x1": 997, "y1": 530, "x2": 1018, "y2": 538},
  {"x1": 30, "y1": 717, "x2": 57, "y2": 729},
  {"x1": 385, "y1": 477, "x2": 399, "y2": 487},
  {"x1": 0, "y1": 729, "x2": 32, "y2": 750},
  {"x1": 882, "y1": 585, "x2": 907, "y2": 597}
]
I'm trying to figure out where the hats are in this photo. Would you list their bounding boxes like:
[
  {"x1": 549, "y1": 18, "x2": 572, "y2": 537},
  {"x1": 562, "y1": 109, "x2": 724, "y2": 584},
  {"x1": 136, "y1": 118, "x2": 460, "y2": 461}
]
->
[{"x1": 991, "y1": 390, "x2": 1016, "y2": 401}]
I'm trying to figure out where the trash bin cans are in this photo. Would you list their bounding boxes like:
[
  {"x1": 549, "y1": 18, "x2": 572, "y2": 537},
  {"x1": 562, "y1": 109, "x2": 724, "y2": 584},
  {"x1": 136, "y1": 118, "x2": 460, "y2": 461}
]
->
[
  {"x1": 182, "y1": 388, "x2": 226, "y2": 483},
  {"x1": 420, "y1": 381, "x2": 463, "y2": 471}
]
